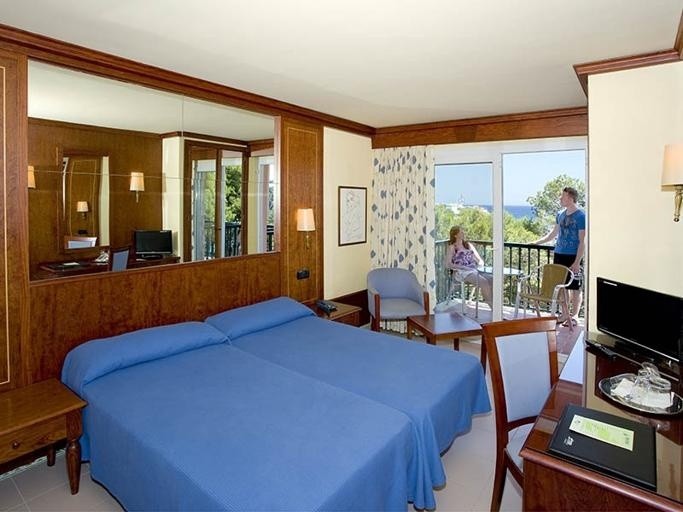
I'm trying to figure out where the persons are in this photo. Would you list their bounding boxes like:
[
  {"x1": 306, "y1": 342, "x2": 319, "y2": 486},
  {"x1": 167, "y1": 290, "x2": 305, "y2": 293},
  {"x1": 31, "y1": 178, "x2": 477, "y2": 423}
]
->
[
  {"x1": 443, "y1": 226, "x2": 507, "y2": 320},
  {"x1": 529, "y1": 187, "x2": 585, "y2": 327}
]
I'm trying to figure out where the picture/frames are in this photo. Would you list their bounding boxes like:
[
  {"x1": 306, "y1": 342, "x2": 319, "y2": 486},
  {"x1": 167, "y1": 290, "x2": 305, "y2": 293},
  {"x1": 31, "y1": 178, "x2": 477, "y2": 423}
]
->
[{"x1": 337, "y1": 185, "x2": 367, "y2": 246}]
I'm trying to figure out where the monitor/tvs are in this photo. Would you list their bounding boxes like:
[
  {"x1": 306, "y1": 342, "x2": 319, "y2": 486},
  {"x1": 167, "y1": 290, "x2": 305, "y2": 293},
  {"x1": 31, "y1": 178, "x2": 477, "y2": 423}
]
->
[
  {"x1": 596, "y1": 276, "x2": 683, "y2": 361},
  {"x1": 135, "y1": 229, "x2": 172, "y2": 258}
]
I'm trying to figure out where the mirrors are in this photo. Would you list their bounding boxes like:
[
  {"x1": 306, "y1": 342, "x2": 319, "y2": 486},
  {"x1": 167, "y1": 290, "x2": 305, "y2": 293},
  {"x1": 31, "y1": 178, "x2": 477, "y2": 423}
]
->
[
  {"x1": 26, "y1": 165, "x2": 36, "y2": 188},
  {"x1": 25, "y1": 57, "x2": 283, "y2": 284},
  {"x1": 54, "y1": 145, "x2": 110, "y2": 253}
]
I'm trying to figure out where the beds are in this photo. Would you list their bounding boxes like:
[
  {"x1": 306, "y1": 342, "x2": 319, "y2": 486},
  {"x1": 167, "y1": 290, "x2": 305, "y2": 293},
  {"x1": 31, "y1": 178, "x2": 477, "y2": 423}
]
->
[
  {"x1": 83, "y1": 316, "x2": 408, "y2": 512},
  {"x1": 228, "y1": 313, "x2": 481, "y2": 490}
]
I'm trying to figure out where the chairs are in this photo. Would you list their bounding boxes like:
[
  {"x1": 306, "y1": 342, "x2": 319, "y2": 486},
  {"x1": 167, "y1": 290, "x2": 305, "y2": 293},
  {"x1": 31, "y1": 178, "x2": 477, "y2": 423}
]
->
[
  {"x1": 444, "y1": 265, "x2": 481, "y2": 315},
  {"x1": 512, "y1": 264, "x2": 575, "y2": 336},
  {"x1": 478, "y1": 315, "x2": 559, "y2": 511},
  {"x1": 366, "y1": 267, "x2": 430, "y2": 333}
]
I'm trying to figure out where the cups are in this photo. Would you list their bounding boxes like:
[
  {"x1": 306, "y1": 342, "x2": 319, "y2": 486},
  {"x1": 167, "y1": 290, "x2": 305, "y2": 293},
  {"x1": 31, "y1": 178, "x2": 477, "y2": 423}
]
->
[{"x1": 631, "y1": 368, "x2": 672, "y2": 409}]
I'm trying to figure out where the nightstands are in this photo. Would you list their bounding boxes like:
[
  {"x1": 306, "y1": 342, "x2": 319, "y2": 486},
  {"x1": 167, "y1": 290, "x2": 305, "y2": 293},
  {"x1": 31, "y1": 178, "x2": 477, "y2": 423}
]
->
[
  {"x1": 0, "y1": 377, "x2": 89, "y2": 497},
  {"x1": 307, "y1": 299, "x2": 363, "y2": 328}
]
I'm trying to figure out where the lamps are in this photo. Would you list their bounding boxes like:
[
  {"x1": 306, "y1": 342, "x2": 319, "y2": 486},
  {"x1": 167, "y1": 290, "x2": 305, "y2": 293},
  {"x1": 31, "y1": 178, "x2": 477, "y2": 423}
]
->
[
  {"x1": 76, "y1": 200, "x2": 89, "y2": 221},
  {"x1": 296, "y1": 208, "x2": 317, "y2": 250},
  {"x1": 660, "y1": 140, "x2": 683, "y2": 222},
  {"x1": 130, "y1": 170, "x2": 145, "y2": 204}
]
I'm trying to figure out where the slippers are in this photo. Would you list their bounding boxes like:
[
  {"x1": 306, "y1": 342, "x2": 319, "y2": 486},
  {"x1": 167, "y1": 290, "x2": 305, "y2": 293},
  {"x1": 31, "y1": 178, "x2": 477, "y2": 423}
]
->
[
  {"x1": 556, "y1": 315, "x2": 564, "y2": 324},
  {"x1": 562, "y1": 319, "x2": 577, "y2": 326}
]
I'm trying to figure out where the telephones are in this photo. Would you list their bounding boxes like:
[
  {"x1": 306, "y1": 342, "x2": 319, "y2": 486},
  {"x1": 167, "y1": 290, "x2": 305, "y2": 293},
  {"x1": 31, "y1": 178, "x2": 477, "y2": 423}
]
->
[{"x1": 317, "y1": 301, "x2": 336, "y2": 314}]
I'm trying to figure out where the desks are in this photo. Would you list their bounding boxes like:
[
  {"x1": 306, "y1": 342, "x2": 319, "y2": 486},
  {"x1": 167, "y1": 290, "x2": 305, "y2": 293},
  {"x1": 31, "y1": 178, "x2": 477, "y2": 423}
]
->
[
  {"x1": 406, "y1": 312, "x2": 487, "y2": 376},
  {"x1": 474, "y1": 266, "x2": 523, "y2": 319}
]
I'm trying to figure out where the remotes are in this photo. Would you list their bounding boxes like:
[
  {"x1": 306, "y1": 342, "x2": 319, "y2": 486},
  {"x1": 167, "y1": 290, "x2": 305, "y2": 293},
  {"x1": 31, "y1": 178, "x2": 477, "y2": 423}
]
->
[{"x1": 585, "y1": 338, "x2": 617, "y2": 357}]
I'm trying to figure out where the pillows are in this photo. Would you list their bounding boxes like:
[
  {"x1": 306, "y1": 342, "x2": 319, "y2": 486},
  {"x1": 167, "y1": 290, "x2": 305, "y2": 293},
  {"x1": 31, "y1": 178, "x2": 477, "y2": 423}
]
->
[
  {"x1": 66, "y1": 321, "x2": 226, "y2": 380},
  {"x1": 205, "y1": 296, "x2": 313, "y2": 338}
]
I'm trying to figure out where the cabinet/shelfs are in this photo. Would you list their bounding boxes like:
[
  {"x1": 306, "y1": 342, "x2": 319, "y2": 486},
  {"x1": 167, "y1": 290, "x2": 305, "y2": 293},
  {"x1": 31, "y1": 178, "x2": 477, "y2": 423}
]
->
[
  {"x1": 30, "y1": 253, "x2": 182, "y2": 281},
  {"x1": 518, "y1": 327, "x2": 683, "y2": 511}
]
